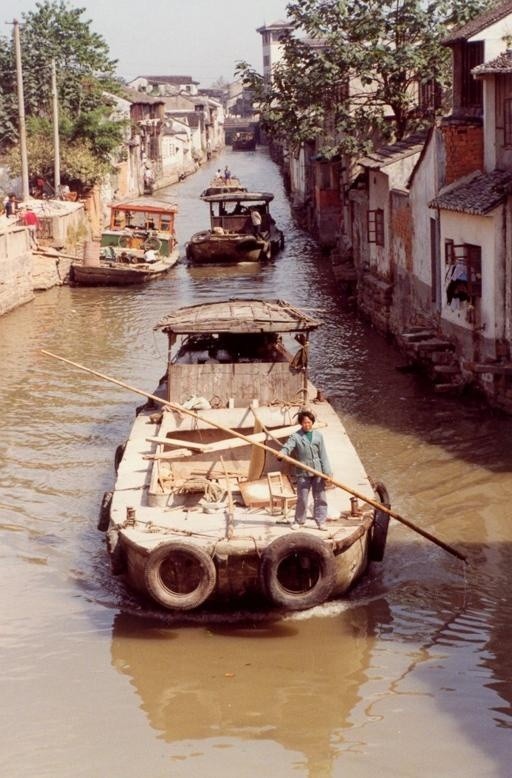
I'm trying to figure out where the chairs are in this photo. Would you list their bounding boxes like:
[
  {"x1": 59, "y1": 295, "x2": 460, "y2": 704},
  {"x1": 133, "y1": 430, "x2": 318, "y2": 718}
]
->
[{"x1": 267, "y1": 471, "x2": 298, "y2": 518}]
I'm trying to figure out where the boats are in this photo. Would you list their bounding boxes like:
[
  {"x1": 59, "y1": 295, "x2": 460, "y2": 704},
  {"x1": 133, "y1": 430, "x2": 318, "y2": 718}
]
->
[
  {"x1": 187, "y1": 177, "x2": 283, "y2": 261},
  {"x1": 232, "y1": 132, "x2": 255, "y2": 151},
  {"x1": 98, "y1": 300, "x2": 389, "y2": 612},
  {"x1": 71, "y1": 199, "x2": 180, "y2": 286}
]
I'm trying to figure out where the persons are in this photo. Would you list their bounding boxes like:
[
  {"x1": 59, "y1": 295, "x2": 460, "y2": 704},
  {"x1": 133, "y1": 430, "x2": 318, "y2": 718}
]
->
[
  {"x1": 274, "y1": 412, "x2": 331, "y2": 530},
  {"x1": 232, "y1": 201, "x2": 246, "y2": 213},
  {"x1": 3, "y1": 177, "x2": 70, "y2": 246},
  {"x1": 145, "y1": 168, "x2": 153, "y2": 187},
  {"x1": 102, "y1": 232, "x2": 162, "y2": 264},
  {"x1": 249, "y1": 206, "x2": 265, "y2": 240},
  {"x1": 215, "y1": 165, "x2": 231, "y2": 185}
]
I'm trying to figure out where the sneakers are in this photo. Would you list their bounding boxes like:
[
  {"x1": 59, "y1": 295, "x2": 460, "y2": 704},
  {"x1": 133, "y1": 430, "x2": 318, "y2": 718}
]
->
[
  {"x1": 319, "y1": 524, "x2": 327, "y2": 531},
  {"x1": 292, "y1": 522, "x2": 299, "y2": 529}
]
